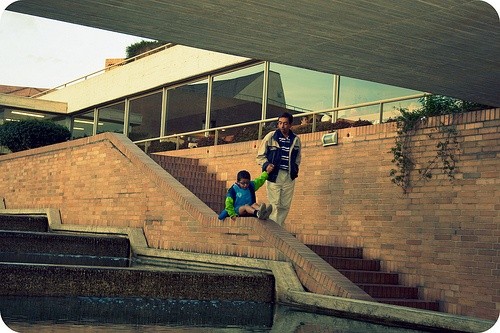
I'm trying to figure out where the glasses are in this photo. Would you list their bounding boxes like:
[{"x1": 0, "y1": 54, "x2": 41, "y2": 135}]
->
[{"x1": 240, "y1": 181, "x2": 250, "y2": 185}]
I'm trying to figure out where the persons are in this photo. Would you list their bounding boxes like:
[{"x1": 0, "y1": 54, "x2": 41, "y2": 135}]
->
[
  {"x1": 256, "y1": 112, "x2": 301, "y2": 227},
  {"x1": 218, "y1": 164, "x2": 273, "y2": 221}
]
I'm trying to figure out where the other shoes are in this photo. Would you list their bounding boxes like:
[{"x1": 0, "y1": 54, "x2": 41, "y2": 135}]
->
[
  {"x1": 264, "y1": 203, "x2": 273, "y2": 220},
  {"x1": 257, "y1": 202, "x2": 266, "y2": 219}
]
